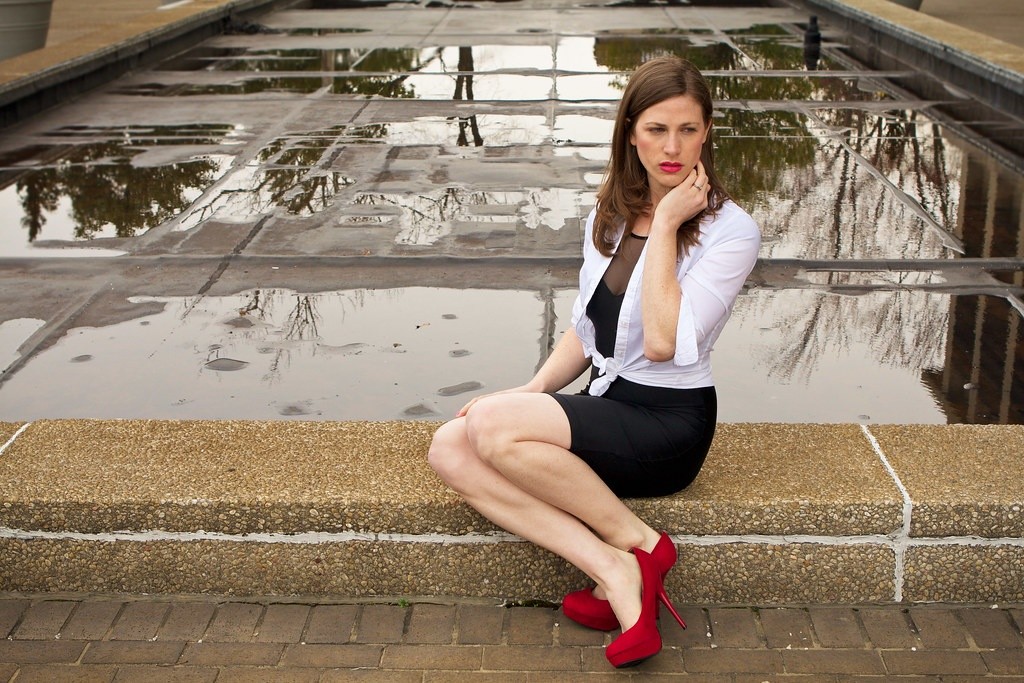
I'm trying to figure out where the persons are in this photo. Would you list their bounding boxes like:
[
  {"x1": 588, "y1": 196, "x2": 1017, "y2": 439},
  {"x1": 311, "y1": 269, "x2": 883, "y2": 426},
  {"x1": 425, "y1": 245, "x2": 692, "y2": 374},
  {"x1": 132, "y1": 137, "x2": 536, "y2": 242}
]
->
[{"x1": 429, "y1": 54, "x2": 761, "y2": 666}]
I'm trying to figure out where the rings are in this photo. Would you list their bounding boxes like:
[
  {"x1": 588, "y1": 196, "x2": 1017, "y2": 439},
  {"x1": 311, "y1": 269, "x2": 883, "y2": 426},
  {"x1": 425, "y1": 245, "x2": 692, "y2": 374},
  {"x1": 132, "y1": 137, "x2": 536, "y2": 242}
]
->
[{"x1": 692, "y1": 183, "x2": 702, "y2": 190}]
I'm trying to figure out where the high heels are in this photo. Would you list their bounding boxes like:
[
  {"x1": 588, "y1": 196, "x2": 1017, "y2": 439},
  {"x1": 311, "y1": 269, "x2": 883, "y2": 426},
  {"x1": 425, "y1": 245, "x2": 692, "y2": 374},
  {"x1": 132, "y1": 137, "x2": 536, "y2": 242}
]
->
[
  {"x1": 604, "y1": 547, "x2": 687, "y2": 669},
  {"x1": 563, "y1": 531, "x2": 676, "y2": 632}
]
